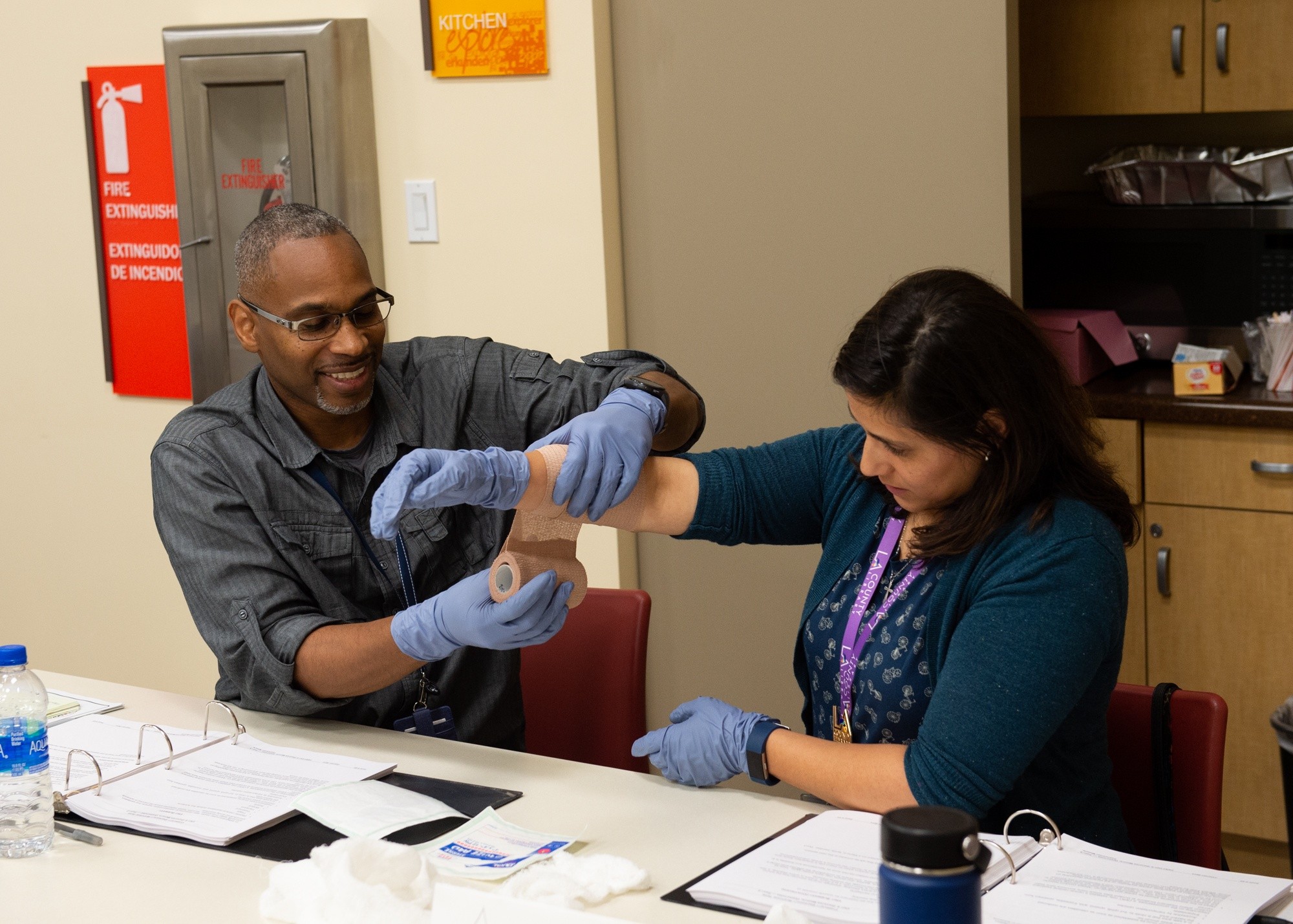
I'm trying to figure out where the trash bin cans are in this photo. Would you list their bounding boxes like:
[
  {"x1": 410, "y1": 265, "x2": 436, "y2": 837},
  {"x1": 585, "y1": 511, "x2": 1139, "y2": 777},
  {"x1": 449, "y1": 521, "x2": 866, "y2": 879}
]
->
[{"x1": 1270, "y1": 702, "x2": 1293, "y2": 880}]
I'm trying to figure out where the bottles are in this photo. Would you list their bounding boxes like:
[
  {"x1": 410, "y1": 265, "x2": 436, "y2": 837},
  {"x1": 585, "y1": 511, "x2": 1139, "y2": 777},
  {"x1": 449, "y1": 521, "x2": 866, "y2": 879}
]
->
[
  {"x1": 879, "y1": 804, "x2": 992, "y2": 924},
  {"x1": 0, "y1": 645, "x2": 55, "y2": 860}
]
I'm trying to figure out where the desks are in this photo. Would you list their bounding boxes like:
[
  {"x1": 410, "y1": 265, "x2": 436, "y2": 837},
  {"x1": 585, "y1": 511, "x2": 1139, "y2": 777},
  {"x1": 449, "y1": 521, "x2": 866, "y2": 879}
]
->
[{"x1": 0, "y1": 669, "x2": 1291, "y2": 924}]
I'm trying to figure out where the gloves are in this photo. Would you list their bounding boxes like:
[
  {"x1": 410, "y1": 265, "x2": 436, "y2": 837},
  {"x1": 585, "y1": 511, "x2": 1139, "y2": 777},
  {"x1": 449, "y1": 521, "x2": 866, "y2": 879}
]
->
[
  {"x1": 370, "y1": 444, "x2": 529, "y2": 541},
  {"x1": 634, "y1": 695, "x2": 765, "y2": 790},
  {"x1": 525, "y1": 388, "x2": 668, "y2": 520},
  {"x1": 391, "y1": 570, "x2": 575, "y2": 664}
]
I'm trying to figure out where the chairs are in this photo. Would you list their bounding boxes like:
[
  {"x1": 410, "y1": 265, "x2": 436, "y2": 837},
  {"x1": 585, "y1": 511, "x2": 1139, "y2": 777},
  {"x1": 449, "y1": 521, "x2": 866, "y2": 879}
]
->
[
  {"x1": 1100, "y1": 675, "x2": 1228, "y2": 867},
  {"x1": 511, "y1": 585, "x2": 650, "y2": 777}
]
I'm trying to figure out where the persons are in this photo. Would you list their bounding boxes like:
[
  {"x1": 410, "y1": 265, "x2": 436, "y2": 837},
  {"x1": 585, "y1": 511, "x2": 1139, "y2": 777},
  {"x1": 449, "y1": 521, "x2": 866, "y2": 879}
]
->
[
  {"x1": 150, "y1": 204, "x2": 705, "y2": 754},
  {"x1": 368, "y1": 267, "x2": 1144, "y2": 834}
]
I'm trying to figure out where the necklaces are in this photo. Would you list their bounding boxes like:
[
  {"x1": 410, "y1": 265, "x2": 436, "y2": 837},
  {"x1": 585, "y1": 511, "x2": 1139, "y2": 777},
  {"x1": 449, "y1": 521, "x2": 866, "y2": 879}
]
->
[{"x1": 883, "y1": 514, "x2": 914, "y2": 603}]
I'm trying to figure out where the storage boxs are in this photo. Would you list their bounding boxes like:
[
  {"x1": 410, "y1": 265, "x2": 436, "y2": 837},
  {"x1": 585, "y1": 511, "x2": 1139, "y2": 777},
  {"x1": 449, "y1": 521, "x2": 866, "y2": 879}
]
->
[
  {"x1": 1173, "y1": 345, "x2": 1237, "y2": 395},
  {"x1": 1028, "y1": 309, "x2": 1138, "y2": 389}
]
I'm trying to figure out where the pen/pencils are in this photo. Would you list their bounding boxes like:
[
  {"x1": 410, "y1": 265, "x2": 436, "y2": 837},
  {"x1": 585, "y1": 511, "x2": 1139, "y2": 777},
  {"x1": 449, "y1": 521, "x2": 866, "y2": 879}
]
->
[{"x1": 54, "y1": 821, "x2": 104, "y2": 846}]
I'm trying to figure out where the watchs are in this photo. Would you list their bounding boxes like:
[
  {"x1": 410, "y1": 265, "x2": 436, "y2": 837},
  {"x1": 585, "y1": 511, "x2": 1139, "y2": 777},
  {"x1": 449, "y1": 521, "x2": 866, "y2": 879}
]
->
[
  {"x1": 745, "y1": 721, "x2": 791, "y2": 786},
  {"x1": 617, "y1": 376, "x2": 670, "y2": 434}
]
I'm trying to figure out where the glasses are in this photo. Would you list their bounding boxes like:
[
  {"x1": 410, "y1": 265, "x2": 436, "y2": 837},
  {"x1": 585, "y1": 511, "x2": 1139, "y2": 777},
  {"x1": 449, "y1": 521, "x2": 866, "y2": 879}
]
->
[{"x1": 237, "y1": 288, "x2": 395, "y2": 343}]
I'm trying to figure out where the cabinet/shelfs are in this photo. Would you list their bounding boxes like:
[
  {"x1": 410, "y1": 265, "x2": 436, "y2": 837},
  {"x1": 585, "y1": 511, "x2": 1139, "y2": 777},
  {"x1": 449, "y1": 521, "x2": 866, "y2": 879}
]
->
[
  {"x1": 1019, "y1": 2, "x2": 1291, "y2": 116},
  {"x1": 1040, "y1": 374, "x2": 1291, "y2": 845}
]
